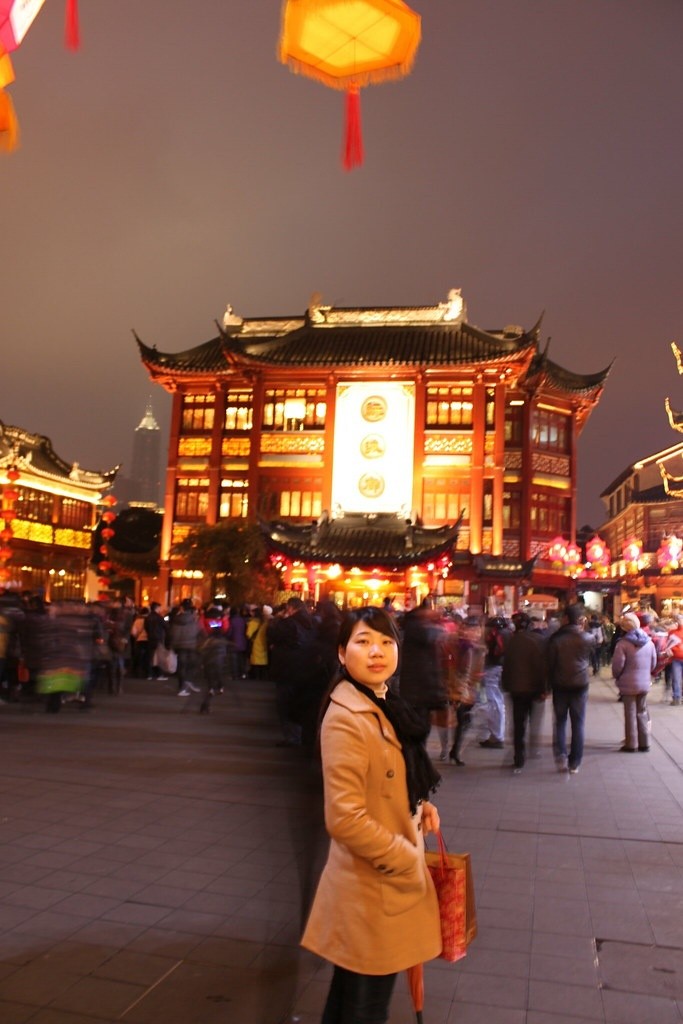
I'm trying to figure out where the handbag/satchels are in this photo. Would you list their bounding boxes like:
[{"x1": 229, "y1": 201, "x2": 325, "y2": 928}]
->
[
  {"x1": 144, "y1": 674, "x2": 153, "y2": 680},
  {"x1": 245, "y1": 640, "x2": 253, "y2": 658},
  {"x1": 156, "y1": 645, "x2": 178, "y2": 674},
  {"x1": 418, "y1": 814, "x2": 467, "y2": 963},
  {"x1": 424, "y1": 828, "x2": 477, "y2": 947}
]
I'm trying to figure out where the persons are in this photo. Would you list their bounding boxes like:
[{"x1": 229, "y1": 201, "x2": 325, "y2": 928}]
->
[
  {"x1": 543, "y1": 605, "x2": 596, "y2": 775},
  {"x1": 612, "y1": 610, "x2": 649, "y2": 752},
  {"x1": 299, "y1": 605, "x2": 443, "y2": 1024},
  {"x1": 497, "y1": 611, "x2": 546, "y2": 770},
  {"x1": 0, "y1": 584, "x2": 683, "y2": 766}
]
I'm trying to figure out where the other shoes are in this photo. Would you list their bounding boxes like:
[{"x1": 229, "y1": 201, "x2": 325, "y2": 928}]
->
[
  {"x1": 439, "y1": 752, "x2": 448, "y2": 761},
  {"x1": 558, "y1": 755, "x2": 569, "y2": 773},
  {"x1": 513, "y1": 768, "x2": 522, "y2": 776},
  {"x1": 569, "y1": 765, "x2": 579, "y2": 774},
  {"x1": 637, "y1": 746, "x2": 651, "y2": 751},
  {"x1": 156, "y1": 675, "x2": 169, "y2": 681},
  {"x1": 620, "y1": 746, "x2": 638, "y2": 752},
  {"x1": 479, "y1": 739, "x2": 504, "y2": 749},
  {"x1": 530, "y1": 751, "x2": 541, "y2": 759}
]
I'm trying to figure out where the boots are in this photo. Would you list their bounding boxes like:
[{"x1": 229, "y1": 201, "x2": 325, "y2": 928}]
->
[{"x1": 671, "y1": 696, "x2": 681, "y2": 706}]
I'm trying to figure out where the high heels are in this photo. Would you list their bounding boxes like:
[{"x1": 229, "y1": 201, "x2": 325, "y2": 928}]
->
[{"x1": 449, "y1": 750, "x2": 465, "y2": 767}]
